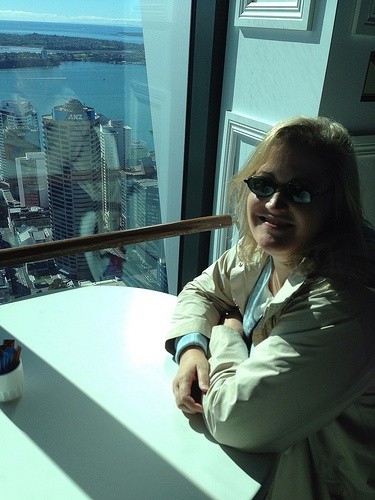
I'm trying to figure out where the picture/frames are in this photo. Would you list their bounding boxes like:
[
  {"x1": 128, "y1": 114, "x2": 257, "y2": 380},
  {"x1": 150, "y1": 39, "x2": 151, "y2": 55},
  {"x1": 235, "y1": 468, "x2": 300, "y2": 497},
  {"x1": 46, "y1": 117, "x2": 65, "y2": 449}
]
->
[
  {"x1": 350, "y1": 0, "x2": 375, "y2": 40},
  {"x1": 359, "y1": 51, "x2": 375, "y2": 102},
  {"x1": 234, "y1": 0, "x2": 316, "y2": 31}
]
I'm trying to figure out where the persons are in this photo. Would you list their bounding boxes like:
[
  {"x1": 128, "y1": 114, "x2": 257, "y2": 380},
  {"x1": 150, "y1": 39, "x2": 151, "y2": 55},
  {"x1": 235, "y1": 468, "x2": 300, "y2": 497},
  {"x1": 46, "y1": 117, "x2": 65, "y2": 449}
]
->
[
  {"x1": 11, "y1": 67, "x2": 124, "y2": 280},
  {"x1": 162, "y1": 114, "x2": 375, "y2": 500}
]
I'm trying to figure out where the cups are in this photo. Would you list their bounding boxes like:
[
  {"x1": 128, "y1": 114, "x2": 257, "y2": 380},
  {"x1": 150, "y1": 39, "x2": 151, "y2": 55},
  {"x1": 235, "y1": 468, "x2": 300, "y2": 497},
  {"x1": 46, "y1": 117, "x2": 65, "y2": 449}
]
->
[{"x1": 0, "y1": 345, "x2": 24, "y2": 402}]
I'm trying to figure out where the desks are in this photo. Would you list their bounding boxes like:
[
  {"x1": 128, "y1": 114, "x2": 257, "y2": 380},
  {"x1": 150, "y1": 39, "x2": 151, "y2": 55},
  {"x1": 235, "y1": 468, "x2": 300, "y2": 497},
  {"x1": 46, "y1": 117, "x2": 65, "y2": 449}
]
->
[{"x1": 0, "y1": 282, "x2": 282, "y2": 500}]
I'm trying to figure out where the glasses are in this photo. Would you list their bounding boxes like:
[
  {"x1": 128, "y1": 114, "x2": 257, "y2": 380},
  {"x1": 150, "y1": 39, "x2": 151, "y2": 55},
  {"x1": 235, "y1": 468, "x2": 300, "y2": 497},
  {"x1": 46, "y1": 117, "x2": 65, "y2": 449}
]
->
[{"x1": 244, "y1": 176, "x2": 313, "y2": 206}]
